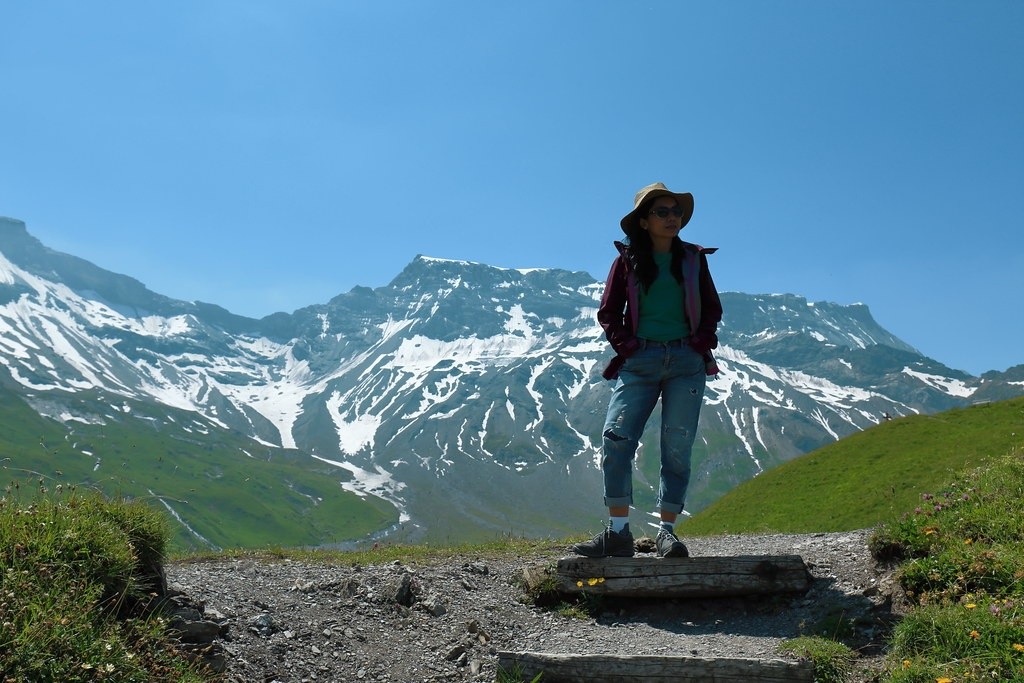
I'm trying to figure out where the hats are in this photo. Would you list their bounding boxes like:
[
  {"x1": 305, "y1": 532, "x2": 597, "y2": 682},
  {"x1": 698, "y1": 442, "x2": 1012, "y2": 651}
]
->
[{"x1": 620, "y1": 182, "x2": 694, "y2": 239}]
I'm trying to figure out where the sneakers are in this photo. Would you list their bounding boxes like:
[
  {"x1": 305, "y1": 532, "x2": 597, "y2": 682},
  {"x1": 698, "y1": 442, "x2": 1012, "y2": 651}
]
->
[
  {"x1": 573, "y1": 520, "x2": 634, "y2": 557},
  {"x1": 655, "y1": 525, "x2": 688, "y2": 558}
]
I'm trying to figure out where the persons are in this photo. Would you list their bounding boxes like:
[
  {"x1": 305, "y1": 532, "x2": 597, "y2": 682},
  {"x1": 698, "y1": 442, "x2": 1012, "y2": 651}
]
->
[{"x1": 572, "y1": 182, "x2": 723, "y2": 559}]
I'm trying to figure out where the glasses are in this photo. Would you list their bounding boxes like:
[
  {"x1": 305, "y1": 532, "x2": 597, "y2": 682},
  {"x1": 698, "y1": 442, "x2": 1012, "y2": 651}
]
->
[{"x1": 648, "y1": 204, "x2": 684, "y2": 219}]
